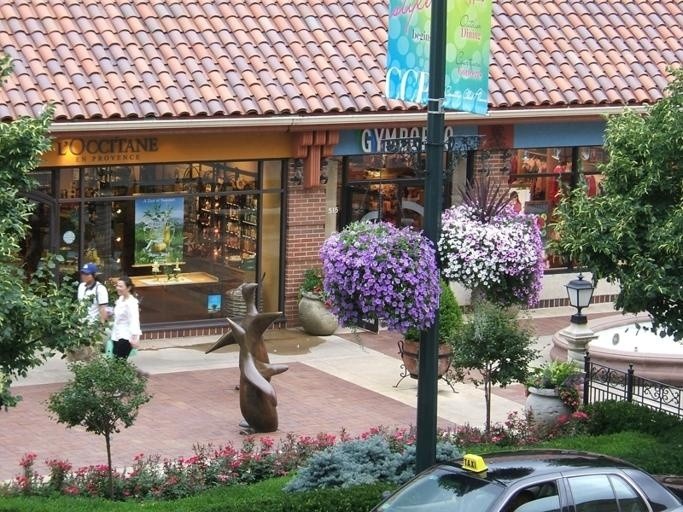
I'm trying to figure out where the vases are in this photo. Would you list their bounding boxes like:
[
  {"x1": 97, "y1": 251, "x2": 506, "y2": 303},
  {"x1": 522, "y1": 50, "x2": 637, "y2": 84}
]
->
[
  {"x1": 472, "y1": 285, "x2": 523, "y2": 321},
  {"x1": 358, "y1": 314, "x2": 390, "y2": 335}
]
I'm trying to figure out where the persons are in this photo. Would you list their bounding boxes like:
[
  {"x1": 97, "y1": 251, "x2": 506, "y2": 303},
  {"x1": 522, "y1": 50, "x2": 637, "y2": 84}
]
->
[
  {"x1": 107, "y1": 277, "x2": 141, "y2": 359},
  {"x1": 509, "y1": 191, "x2": 522, "y2": 214},
  {"x1": 75, "y1": 262, "x2": 107, "y2": 326}
]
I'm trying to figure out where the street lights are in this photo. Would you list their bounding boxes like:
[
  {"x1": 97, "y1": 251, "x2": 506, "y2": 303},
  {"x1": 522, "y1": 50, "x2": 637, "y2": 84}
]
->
[{"x1": 559, "y1": 272, "x2": 599, "y2": 405}]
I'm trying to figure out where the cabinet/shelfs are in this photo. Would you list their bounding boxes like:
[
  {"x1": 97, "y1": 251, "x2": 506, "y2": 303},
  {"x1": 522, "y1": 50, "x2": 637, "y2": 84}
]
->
[
  {"x1": 27, "y1": 159, "x2": 259, "y2": 261},
  {"x1": 128, "y1": 271, "x2": 220, "y2": 323}
]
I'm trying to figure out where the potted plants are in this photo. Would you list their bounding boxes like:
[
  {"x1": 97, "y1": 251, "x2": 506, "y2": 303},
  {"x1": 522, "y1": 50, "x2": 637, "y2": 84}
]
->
[
  {"x1": 402, "y1": 277, "x2": 462, "y2": 380},
  {"x1": 98, "y1": 277, "x2": 122, "y2": 352},
  {"x1": 524, "y1": 357, "x2": 592, "y2": 439},
  {"x1": 297, "y1": 269, "x2": 339, "y2": 336}
]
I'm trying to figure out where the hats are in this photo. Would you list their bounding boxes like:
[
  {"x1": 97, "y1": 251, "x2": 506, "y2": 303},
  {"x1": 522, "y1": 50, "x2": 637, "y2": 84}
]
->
[{"x1": 78, "y1": 263, "x2": 97, "y2": 274}]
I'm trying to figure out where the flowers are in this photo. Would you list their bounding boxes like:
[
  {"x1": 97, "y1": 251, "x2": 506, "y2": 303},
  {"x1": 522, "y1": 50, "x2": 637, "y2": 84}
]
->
[
  {"x1": 318, "y1": 219, "x2": 441, "y2": 336},
  {"x1": 437, "y1": 171, "x2": 550, "y2": 313}
]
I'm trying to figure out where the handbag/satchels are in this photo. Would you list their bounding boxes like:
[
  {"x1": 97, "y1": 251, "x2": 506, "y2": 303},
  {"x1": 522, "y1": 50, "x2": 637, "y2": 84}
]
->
[{"x1": 105, "y1": 340, "x2": 136, "y2": 359}]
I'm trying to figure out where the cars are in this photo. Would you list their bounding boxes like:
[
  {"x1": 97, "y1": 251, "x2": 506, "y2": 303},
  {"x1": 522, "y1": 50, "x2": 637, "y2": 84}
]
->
[{"x1": 367, "y1": 449, "x2": 683, "y2": 512}]
